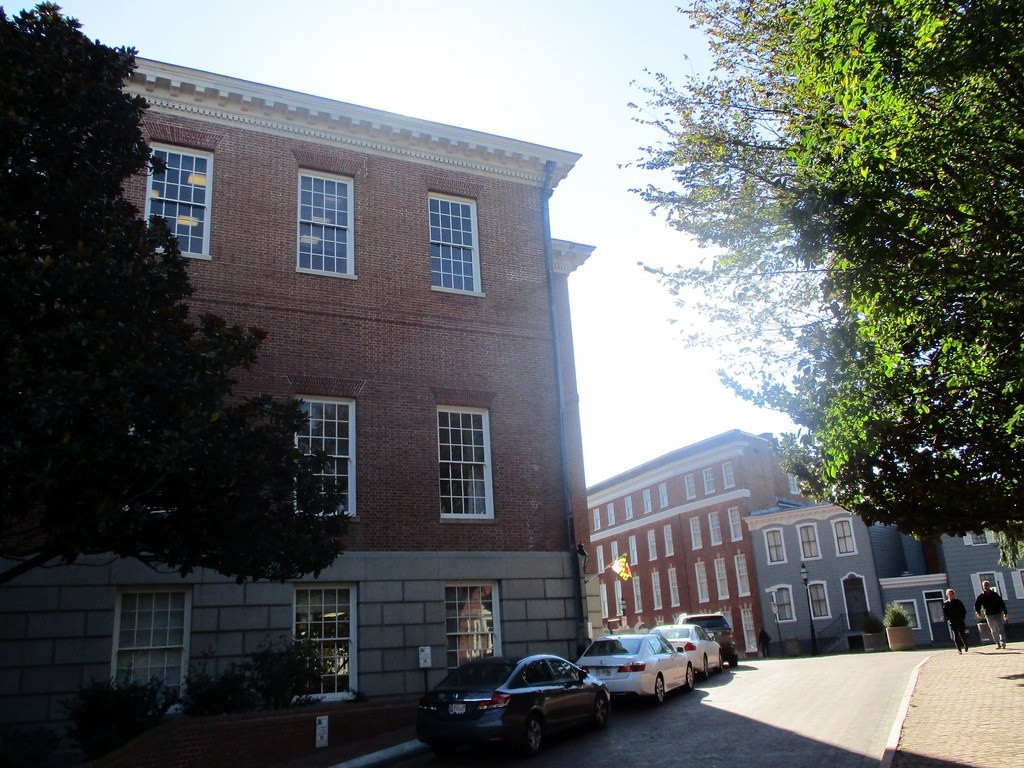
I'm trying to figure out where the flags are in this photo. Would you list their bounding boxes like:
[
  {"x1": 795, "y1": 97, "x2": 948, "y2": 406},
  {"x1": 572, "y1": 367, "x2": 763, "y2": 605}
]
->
[{"x1": 610, "y1": 553, "x2": 632, "y2": 581}]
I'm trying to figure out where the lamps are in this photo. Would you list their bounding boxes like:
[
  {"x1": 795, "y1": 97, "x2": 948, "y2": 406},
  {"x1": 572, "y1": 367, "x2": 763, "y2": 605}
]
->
[{"x1": 576, "y1": 541, "x2": 589, "y2": 558}]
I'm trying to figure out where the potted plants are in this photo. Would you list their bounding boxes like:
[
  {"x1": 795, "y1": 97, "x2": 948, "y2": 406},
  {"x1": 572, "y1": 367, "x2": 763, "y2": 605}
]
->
[
  {"x1": 881, "y1": 600, "x2": 916, "y2": 651},
  {"x1": 859, "y1": 608, "x2": 887, "y2": 652},
  {"x1": 974, "y1": 603, "x2": 1004, "y2": 644}
]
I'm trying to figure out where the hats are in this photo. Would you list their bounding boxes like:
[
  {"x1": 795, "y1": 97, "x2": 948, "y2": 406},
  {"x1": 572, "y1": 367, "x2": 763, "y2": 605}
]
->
[{"x1": 982, "y1": 580, "x2": 990, "y2": 586}]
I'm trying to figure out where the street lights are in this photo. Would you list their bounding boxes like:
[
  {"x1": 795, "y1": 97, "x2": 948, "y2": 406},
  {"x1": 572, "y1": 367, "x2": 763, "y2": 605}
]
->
[
  {"x1": 620, "y1": 596, "x2": 627, "y2": 616},
  {"x1": 800, "y1": 563, "x2": 820, "y2": 656}
]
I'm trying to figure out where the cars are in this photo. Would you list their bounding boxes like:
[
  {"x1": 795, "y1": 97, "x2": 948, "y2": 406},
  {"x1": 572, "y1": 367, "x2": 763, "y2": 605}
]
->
[
  {"x1": 416, "y1": 652, "x2": 611, "y2": 760},
  {"x1": 575, "y1": 634, "x2": 694, "y2": 707},
  {"x1": 649, "y1": 624, "x2": 723, "y2": 682}
]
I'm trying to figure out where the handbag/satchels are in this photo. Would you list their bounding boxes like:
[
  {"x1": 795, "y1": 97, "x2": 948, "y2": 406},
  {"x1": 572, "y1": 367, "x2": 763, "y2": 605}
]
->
[{"x1": 766, "y1": 632, "x2": 771, "y2": 641}]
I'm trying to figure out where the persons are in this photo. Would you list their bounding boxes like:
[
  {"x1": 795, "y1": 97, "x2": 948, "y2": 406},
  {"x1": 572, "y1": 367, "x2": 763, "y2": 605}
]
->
[
  {"x1": 942, "y1": 589, "x2": 968, "y2": 655},
  {"x1": 758, "y1": 628, "x2": 771, "y2": 658},
  {"x1": 974, "y1": 581, "x2": 1008, "y2": 649}
]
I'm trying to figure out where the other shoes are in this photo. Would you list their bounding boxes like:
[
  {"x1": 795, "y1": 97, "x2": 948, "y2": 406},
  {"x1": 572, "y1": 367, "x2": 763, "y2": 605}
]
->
[
  {"x1": 964, "y1": 644, "x2": 968, "y2": 652},
  {"x1": 1002, "y1": 643, "x2": 1005, "y2": 649},
  {"x1": 958, "y1": 650, "x2": 962, "y2": 654},
  {"x1": 996, "y1": 646, "x2": 1001, "y2": 650}
]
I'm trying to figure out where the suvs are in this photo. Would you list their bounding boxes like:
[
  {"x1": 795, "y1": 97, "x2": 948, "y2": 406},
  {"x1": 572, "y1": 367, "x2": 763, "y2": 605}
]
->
[{"x1": 676, "y1": 612, "x2": 738, "y2": 668}]
